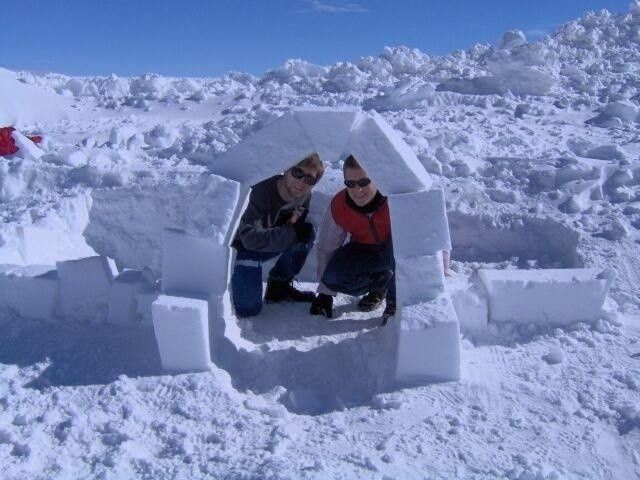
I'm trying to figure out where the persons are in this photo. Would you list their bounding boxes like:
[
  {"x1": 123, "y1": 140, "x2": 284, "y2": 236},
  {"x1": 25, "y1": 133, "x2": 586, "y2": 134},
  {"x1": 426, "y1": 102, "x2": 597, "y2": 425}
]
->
[
  {"x1": 307, "y1": 154, "x2": 453, "y2": 317},
  {"x1": 228, "y1": 153, "x2": 325, "y2": 321}
]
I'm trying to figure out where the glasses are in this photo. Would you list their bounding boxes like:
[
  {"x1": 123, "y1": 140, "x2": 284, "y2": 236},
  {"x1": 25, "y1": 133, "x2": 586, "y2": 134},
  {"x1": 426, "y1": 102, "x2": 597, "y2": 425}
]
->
[
  {"x1": 291, "y1": 166, "x2": 317, "y2": 186},
  {"x1": 344, "y1": 177, "x2": 372, "y2": 188}
]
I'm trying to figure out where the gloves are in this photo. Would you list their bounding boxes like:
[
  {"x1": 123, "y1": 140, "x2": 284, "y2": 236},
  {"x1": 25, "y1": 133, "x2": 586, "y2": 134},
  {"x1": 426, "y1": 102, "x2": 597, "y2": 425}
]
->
[
  {"x1": 294, "y1": 222, "x2": 315, "y2": 245},
  {"x1": 309, "y1": 292, "x2": 334, "y2": 319}
]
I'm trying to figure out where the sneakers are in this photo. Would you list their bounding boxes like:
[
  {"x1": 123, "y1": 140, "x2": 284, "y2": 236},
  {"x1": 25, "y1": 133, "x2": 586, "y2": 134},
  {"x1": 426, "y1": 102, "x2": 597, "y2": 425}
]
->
[
  {"x1": 357, "y1": 271, "x2": 396, "y2": 324},
  {"x1": 264, "y1": 277, "x2": 317, "y2": 305}
]
